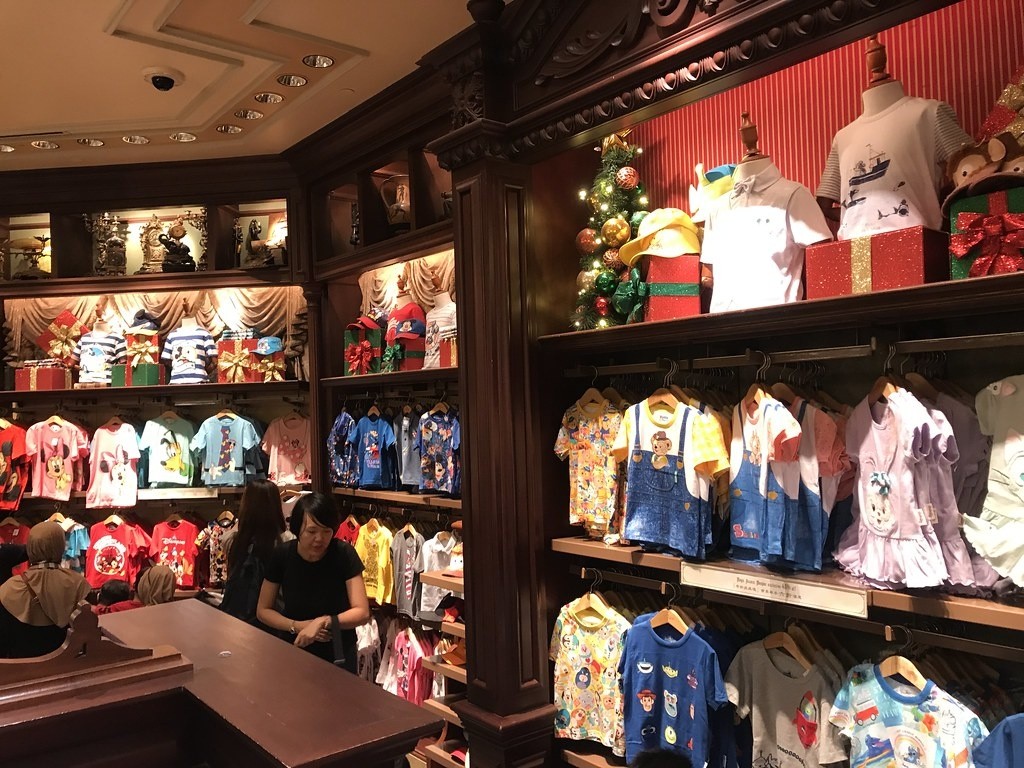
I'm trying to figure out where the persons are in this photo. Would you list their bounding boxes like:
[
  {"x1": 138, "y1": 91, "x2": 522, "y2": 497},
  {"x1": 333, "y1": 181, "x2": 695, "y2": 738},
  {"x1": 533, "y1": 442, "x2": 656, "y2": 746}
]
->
[
  {"x1": 91, "y1": 567, "x2": 149, "y2": 615},
  {"x1": 136, "y1": 566, "x2": 176, "y2": 608},
  {"x1": 812, "y1": 77, "x2": 978, "y2": 241},
  {"x1": 216, "y1": 478, "x2": 287, "y2": 631},
  {"x1": 424, "y1": 290, "x2": 461, "y2": 371},
  {"x1": 385, "y1": 290, "x2": 427, "y2": 352},
  {"x1": 71, "y1": 319, "x2": 127, "y2": 386},
  {"x1": 699, "y1": 150, "x2": 833, "y2": 313},
  {"x1": 162, "y1": 316, "x2": 219, "y2": 386},
  {"x1": 0, "y1": 521, "x2": 90, "y2": 659},
  {"x1": 257, "y1": 492, "x2": 370, "y2": 674},
  {"x1": 96, "y1": 579, "x2": 134, "y2": 607}
]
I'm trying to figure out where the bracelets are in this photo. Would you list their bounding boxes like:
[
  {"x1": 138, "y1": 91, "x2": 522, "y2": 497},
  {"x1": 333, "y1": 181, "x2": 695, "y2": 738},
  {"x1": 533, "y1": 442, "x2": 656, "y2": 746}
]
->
[{"x1": 288, "y1": 621, "x2": 299, "y2": 635}]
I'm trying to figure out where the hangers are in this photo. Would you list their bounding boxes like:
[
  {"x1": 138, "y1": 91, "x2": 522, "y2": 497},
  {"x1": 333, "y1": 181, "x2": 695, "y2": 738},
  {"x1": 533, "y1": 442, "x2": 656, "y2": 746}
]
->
[
  {"x1": 878, "y1": 624, "x2": 968, "y2": 689},
  {"x1": 282, "y1": 399, "x2": 308, "y2": 422},
  {"x1": 764, "y1": 619, "x2": 843, "y2": 668},
  {"x1": 103, "y1": 507, "x2": 137, "y2": 529},
  {"x1": 652, "y1": 357, "x2": 732, "y2": 406},
  {"x1": 741, "y1": 351, "x2": 842, "y2": 412},
  {"x1": 158, "y1": 406, "x2": 184, "y2": 420},
  {"x1": 44, "y1": 405, "x2": 74, "y2": 426},
  {"x1": 217, "y1": 401, "x2": 241, "y2": 419},
  {"x1": 341, "y1": 500, "x2": 456, "y2": 543},
  {"x1": 337, "y1": 391, "x2": 449, "y2": 419},
  {"x1": 47, "y1": 499, "x2": 74, "y2": 521},
  {"x1": 214, "y1": 500, "x2": 238, "y2": 526},
  {"x1": 105, "y1": 407, "x2": 138, "y2": 426},
  {"x1": 0, "y1": 511, "x2": 31, "y2": 527},
  {"x1": 164, "y1": 506, "x2": 195, "y2": 526},
  {"x1": 867, "y1": 341, "x2": 953, "y2": 403},
  {"x1": 567, "y1": 567, "x2": 752, "y2": 635},
  {"x1": 581, "y1": 366, "x2": 650, "y2": 407},
  {"x1": 0, "y1": 410, "x2": 24, "y2": 429},
  {"x1": 372, "y1": 607, "x2": 452, "y2": 652}
]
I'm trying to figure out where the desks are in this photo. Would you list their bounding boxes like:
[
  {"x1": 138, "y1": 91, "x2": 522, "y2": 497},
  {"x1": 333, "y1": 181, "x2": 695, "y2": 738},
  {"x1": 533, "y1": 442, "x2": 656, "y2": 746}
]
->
[{"x1": 98, "y1": 599, "x2": 444, "y2": 768}]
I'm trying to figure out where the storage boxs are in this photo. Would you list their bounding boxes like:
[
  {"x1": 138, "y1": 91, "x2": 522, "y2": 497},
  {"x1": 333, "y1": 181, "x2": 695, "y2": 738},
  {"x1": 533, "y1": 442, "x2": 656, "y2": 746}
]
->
[
  {"x1": 384, "y1": 332, "x2": 426, "y2": 370},
  {"x1": 112, "y1": 365, "x2": 158, "y2": 387},
  {"x1": 14, "y1": 369, "x2": 65, "y2": 391},
  {"x1": 126, "y1": 333, "x2": 159, "y2": 365},
  {"x1": 35, "y1": 310, "x2": 90, "y2": 367},
  {"x1": 217, "y1": 340, "x2": 284, "y2": 382},
  {"x1": 950, "y1": 188, "x2": 1024, "y2": 280},
  {"x1": 638, "y1": 255, "x2": 700, "y2": 323},
  {"x1": 805, "y1": 226, "x2": 951, "y2": 300},
  {"x1": 440, "y1": 338, "x2": 457, "y2": 368},
  {"x1": 344, "y1": 329, "x2": 398, "y2": 376}
]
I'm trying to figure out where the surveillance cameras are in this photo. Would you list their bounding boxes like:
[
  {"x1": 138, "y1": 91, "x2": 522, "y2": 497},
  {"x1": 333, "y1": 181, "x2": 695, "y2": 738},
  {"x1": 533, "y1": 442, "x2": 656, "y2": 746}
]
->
[{"x1": 144, "y1": 68, "x2": 183, "y2": 92}]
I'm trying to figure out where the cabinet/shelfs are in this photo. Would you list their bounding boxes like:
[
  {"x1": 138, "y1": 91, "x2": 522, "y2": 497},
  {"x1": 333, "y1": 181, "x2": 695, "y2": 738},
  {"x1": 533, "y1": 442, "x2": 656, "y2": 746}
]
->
[
  {"x1": 537, "y1": 261, "x2": 1024, "y2": 768},
  {"x1": 313, "y1": 227, "x2": 468, "y2": 768},
  {"x1": 0, "y1": 275, "x2": 313, "y2": 598}
]
